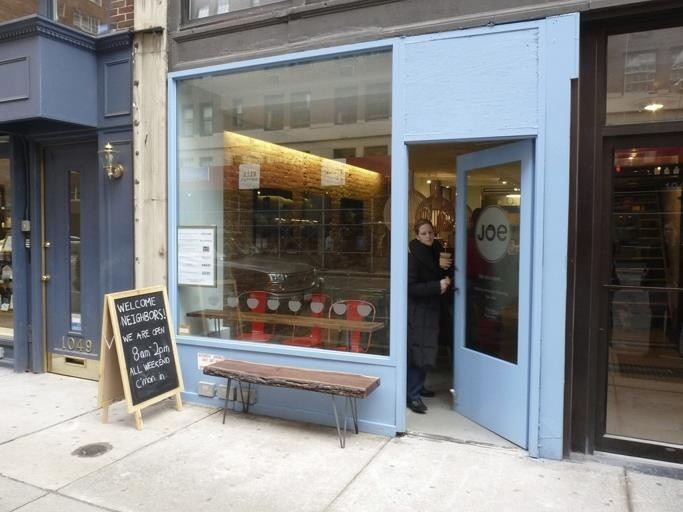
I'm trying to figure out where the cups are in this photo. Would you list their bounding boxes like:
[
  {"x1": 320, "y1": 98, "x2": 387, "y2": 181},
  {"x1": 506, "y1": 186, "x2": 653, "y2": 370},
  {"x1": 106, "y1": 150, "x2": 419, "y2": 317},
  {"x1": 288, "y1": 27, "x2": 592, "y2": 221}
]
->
[{"x1": 439, "y1": 252, "x2": 451, "y2": 269}]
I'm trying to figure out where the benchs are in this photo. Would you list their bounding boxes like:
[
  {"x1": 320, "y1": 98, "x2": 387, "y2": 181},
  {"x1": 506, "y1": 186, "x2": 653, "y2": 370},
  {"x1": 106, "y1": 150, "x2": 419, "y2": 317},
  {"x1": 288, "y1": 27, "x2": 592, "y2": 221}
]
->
[{"x1": 202, "y1": 358, "x2": 381, "y2": 448}]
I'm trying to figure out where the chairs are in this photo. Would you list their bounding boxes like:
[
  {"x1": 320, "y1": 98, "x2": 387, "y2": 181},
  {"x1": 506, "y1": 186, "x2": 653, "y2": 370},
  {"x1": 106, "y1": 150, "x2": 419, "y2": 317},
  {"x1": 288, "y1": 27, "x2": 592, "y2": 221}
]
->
[{"x1": 185, "y1": 290, "x2": 387, "y2": 355}]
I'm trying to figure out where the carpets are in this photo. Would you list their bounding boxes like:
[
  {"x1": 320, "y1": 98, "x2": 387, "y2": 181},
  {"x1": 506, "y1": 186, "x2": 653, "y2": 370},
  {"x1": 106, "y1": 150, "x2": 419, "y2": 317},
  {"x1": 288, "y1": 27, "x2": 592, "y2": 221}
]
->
[{"x1": 615, "y1": 351, "x2": 683, "y2": 385}]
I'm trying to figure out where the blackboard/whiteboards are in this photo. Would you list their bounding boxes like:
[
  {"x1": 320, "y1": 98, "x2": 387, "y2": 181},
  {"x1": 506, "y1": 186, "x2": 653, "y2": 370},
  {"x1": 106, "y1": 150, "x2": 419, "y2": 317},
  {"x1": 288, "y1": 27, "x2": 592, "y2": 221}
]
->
[{"x1": 108, "y1": 285, "x2": 184, "y2": 414}]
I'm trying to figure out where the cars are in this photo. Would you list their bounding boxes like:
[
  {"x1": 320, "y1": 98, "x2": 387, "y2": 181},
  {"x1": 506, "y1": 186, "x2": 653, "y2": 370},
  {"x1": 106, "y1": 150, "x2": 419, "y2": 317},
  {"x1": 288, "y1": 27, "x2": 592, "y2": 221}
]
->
[
  {"x1": 180, "y1": 222, "x2": 321, "y2": 332},
  {"x1": 68, "y1": 233, "x2": 80, "y2": 292}
]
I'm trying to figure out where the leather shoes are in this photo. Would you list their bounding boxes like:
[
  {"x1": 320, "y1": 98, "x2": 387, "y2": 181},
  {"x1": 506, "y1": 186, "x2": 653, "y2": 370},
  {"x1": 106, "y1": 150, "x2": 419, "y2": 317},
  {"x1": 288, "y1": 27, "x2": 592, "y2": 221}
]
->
[
  {"x1": 421, "y1": 388, "x2": 434, "y2": 397},
  {"x1": 407, "y1": 399, "x2": 427, "y2": 411}
]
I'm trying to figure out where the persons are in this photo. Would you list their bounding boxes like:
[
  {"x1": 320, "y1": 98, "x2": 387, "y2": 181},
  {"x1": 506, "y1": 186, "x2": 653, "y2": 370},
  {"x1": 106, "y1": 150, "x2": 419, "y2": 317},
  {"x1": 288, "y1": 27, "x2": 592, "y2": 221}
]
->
[{"x1": 406, "y1": 219, "x2": 453, "y2": 412}]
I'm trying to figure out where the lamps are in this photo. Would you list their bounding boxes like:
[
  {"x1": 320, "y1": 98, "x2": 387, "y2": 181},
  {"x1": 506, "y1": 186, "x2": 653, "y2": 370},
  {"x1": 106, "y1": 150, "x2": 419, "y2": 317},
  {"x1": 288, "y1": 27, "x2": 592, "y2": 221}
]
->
[{"x1": 97, "y1": 141, "x2": 124, "y2": 181}]
